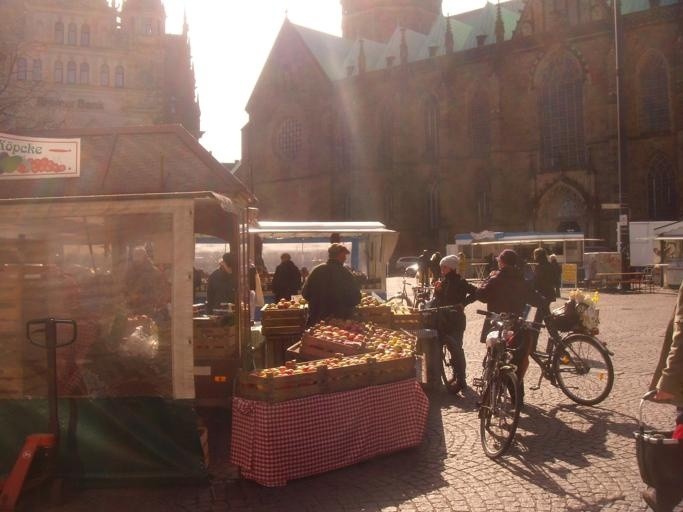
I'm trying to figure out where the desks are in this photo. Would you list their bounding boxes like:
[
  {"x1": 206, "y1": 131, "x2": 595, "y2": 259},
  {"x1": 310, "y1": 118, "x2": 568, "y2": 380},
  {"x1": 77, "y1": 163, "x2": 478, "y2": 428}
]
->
[
  {"x1": 596, "y1": 272, "x2": 656, "y2": 292},
  {"x1": 527, "y1": 263, "x2": 539, "y2": 271},
  {"x1": 232, "y1": 378, "x2": 418, "y2": 487},
  {"x1": 470, "y1": 263, "x2": 490, "y2": 286},
  {"x1": 407, "y1": 328, "x2": 441, "y2": 394}
]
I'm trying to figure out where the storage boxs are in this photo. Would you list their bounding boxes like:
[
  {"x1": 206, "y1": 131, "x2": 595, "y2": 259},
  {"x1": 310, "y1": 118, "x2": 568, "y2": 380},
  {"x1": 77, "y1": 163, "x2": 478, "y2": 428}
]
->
[{"x1": 194, "y1": 314, "x2": 237, "y2": 361}]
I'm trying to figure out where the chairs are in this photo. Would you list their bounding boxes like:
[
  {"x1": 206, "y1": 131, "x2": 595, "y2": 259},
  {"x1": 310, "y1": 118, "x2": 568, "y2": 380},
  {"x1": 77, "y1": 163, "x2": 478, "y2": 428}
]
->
[{"x1": 561, "y1": 264, "x2": 578, "y2": 288}]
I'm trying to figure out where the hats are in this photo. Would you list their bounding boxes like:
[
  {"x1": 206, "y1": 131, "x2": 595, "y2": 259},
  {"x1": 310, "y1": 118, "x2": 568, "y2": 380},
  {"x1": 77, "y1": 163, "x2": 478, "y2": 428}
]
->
[
  {"x1": 438, "y1": 254, "x2": 459, "y2": 273},
  {"x1": 328, "y1": 243, "x2": 350, "y2": 256}
]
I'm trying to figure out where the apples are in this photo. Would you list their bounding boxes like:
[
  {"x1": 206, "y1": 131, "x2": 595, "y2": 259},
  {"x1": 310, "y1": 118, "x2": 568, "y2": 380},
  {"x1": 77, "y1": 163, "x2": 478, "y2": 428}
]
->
[
  {"x1": 266, "y1": 298, "x2": 308, "y2": 310},
  {"x1": 357, "y1": 290, "x2": 419, "y2": 315},
  {"x1": 16, "y1": 158, "x2": 65, "y2": 174},
  {"x1": 249, "y1": 320, "x2": 414, "y2": 379}
]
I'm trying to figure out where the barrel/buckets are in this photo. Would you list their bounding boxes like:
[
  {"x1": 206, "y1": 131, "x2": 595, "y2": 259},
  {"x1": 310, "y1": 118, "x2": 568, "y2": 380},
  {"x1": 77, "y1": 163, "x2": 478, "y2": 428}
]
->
[{"x1": 409, "y1": 322, "x2": 443, "y2": 393}]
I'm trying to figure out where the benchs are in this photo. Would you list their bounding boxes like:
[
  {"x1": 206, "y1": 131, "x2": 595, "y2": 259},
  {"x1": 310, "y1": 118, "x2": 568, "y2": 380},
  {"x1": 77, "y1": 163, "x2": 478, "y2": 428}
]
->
[{"x1": 591, "y1": 280, "x2": 661, "y2": 293}]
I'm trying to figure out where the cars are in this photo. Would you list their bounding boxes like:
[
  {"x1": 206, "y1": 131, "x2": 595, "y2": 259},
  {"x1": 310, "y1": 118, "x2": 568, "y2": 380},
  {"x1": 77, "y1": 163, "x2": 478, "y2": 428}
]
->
[{"x1": 395, "y1": 255, "x2": 419, "y2": 278}]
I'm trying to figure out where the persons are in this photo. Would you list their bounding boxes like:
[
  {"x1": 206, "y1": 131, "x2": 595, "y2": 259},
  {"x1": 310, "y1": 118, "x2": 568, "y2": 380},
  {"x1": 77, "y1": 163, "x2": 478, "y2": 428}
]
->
[
  {"x1": 193, "y1": 250, "x2": 301, "y2": 310},
  {"x1": 416, "y1": 248, "x2": 562, "y2": 389},
  {"x1": 126, "y1": 247, "x2": 168, "y2": 313},
  {"x1": 301, "y1": 242, "x2": 361, "y2": 328},
  {"x1": 641, "y1": 279, "x2": 683, "y2": 512}
]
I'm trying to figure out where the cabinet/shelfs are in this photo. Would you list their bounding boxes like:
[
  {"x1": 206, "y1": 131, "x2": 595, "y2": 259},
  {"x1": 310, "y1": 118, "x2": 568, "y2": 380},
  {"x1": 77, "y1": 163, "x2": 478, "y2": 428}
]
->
[{"x1": 265, "y1": 334, "x2": 303, "y2": 368}]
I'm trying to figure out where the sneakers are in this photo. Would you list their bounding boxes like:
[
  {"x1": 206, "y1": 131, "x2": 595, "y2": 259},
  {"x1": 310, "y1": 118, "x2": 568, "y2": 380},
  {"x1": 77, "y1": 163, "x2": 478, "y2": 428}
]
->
[
  {"x1": 445, "y1": 377, "x2": 466, "y2": 393},
  {"x1": 642, "y1": 488, "x2": 671, "y2": 511}
]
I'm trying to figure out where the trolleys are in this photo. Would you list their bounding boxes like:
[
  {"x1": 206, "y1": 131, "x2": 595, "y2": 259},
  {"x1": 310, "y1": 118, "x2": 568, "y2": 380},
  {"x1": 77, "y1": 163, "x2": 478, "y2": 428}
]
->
[{"x1": 0, "y1": 319, "x2": 79, "y2": 512}]
